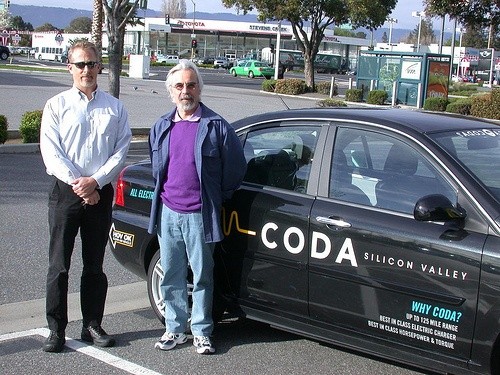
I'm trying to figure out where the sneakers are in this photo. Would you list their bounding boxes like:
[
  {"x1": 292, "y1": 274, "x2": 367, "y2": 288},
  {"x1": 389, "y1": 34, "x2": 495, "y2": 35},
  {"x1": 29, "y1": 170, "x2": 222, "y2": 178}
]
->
[
  {"x1": 155, "y1": 331, "x2": 187, "y2": 351},
  {"x1": 193, "y1": 335, "x2": 215, "y2": 354},
  {"x1": 81, "y1": 320, "x2": 115, "y2": 347},
  {"x1": 43, "y1": 330, "x2": 65, "y2": 352}
]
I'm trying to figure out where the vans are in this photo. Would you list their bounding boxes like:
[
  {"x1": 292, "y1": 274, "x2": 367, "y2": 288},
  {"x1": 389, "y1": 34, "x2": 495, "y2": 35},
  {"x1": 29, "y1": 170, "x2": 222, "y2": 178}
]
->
[
  {"x1": 225, "y1": 54, "x2": 237, "y2": 66},
  {"x1": 34, "y1": 47, "x2": 63, "y2": 62},
  {"x1": 201, "y1": 56, "x2": 215, "y2": 64},
  {"x1": 156, "y1": 55, "x2": 179, "y2": 65}
]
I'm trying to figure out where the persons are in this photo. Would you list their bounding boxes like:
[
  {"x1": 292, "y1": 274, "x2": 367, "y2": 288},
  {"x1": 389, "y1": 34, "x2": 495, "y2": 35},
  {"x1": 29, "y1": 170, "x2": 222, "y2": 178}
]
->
[
  {"x1": 147, "y1": 62, "x2": 247, "y2": 355},
  {"x1": 39, "y1": 40, "x2": 131, "y2": 352}
]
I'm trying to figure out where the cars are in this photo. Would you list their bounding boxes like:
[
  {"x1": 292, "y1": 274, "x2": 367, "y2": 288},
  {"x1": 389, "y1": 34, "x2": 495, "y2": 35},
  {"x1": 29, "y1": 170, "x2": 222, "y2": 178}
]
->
[
  {"x1": 6, "y1": 46, "x2": 37, "y2": 55},
  {"x1": 229, "y1": 60, "x2": 275, "y2": 80},
  {"x1": 452, "y1": 74, "x2": 481, "y2": 83},
  {"x1": 213, "y1": 56, "x2": 230, "y2": 69},
  {"x1": 189, "y1": 57, "x2": 201, "y2": 63},
  {"x1": 107, "y1": 107, "x2": 499, "y2": 374},
  {"x1": 0, "y1": 45, "x2": 9, "y2": 60},
  {"x1": 101, "y1": 47, "x2": 108, "y2": 58}
]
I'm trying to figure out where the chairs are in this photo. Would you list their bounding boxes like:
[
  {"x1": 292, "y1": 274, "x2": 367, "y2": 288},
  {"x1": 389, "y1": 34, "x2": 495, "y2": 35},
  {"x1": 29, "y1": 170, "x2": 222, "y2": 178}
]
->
[
  {"x1": 375, "y1": 143, "x2": 418, "y2": 203},
  {"x1": 333, "y1": 149, "x2": 372, "y2": 207}
]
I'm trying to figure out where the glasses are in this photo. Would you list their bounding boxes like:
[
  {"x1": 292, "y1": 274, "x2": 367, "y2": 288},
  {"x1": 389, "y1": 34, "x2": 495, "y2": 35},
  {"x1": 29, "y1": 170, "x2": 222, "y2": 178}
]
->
[
  {"x1": 168, "y1": 81, "x2": 199, "y2": 91},
  {"x1": 71, "y1": 61, "x2": 98, "y2": 69}
]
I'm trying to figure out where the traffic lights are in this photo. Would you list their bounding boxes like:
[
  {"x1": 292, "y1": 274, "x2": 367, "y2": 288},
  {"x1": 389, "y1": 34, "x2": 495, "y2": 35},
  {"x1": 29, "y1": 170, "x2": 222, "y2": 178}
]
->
[
  {"x1": 192, "y1": 39, "x2": 197, "y2": 48},
  {"x1": 270, "y1": 44, "x2": 274, "y2": 54},
  {"x1": 165, "y1": 14, "x2": 169, "y2": 24},
  {"x1": 5, "y1": 0, "x2": 10, "y2": 8}
]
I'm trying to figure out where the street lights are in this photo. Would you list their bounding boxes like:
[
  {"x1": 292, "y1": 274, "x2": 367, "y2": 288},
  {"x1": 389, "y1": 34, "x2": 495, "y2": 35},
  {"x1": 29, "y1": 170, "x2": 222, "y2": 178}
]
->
[
  {"x1": 385, "y1": 17, "x2": 397, "y2": 51},
  {"x1": 412, "y1": 11, "x2": 427, "y2": 50}
]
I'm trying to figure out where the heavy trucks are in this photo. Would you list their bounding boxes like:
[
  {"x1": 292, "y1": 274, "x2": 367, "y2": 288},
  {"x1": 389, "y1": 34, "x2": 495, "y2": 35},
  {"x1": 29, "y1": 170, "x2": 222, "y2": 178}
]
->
[{"x1": 261, "y1": 47, "x2": 350, "y2": 75}]
